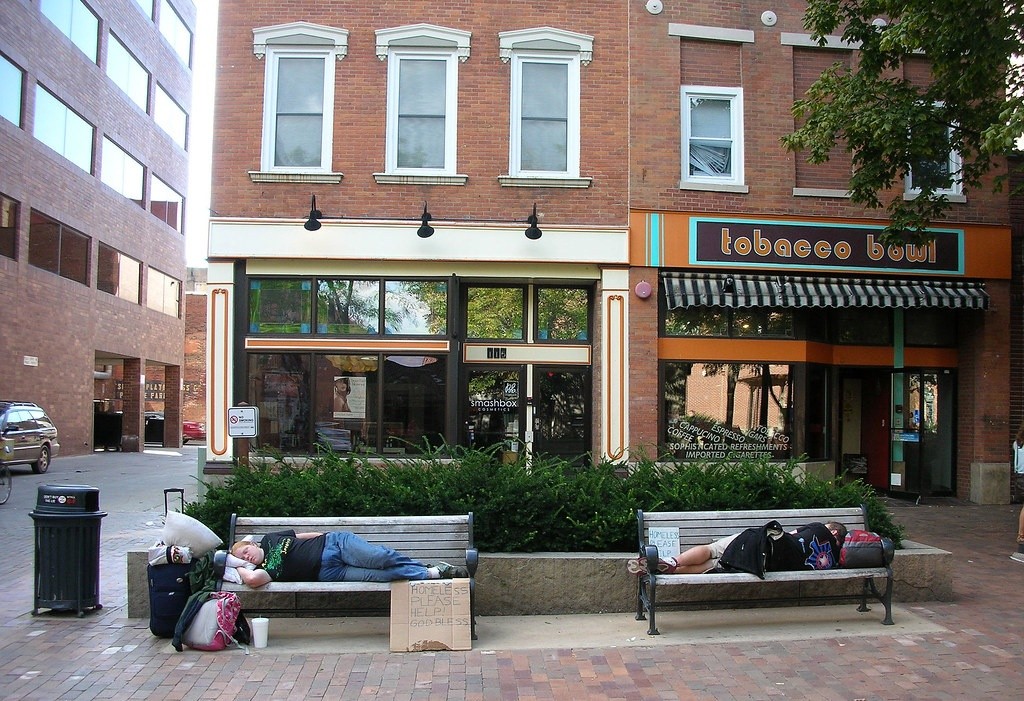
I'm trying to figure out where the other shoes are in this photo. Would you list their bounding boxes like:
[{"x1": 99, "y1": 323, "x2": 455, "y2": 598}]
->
[
  {"x1": 638, "y1": 556, "x2": 679, "y2": 573},
  {"x1": 1009, "y1": 552, "x2": 1024, "y2": 563},
  {"x1": 449, "y1": 566, "x2": 469, "y2": 578},
  {"x1": 437, "y1": 561, "x2": 455, "y2": 579},
  {"x1": 626, "y1": 559, "x2": 649, "y2": 575}
]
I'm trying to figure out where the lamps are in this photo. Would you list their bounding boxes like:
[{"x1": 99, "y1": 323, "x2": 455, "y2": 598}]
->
[
  {"x1": 304, "y1": 195, "x2": 322, "y2": 231},
  {"x1": 526, "y1": 203, "x2": 542, "y2": 239},
  {"x1": 418, "y1": 205, "x2": 434, "y2": 237}
]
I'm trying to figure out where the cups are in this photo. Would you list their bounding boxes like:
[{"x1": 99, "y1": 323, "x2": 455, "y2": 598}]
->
[{"x1": 252, "y1": 618, "x2": 269, "y2": 648}]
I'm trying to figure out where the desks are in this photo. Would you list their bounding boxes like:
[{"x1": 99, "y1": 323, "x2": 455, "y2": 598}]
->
[{"x1": 360, "y1": 446, "x2": 405, "y2": 457}]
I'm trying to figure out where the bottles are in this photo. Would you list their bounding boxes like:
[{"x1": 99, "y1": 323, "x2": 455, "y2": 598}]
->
[
  {"x1": 383, "y1": 434, "x2": 404, "y2": 448},
  {"x1": 352, "y1": 433, "x2": 376, "y2": 448}
]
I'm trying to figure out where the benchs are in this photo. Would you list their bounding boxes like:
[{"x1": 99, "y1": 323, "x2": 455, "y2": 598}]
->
[
  {"x1": 636, "y1": 508, "x2": 895, "y2": 637},
  {"x1": 224, "y1": 513, "x2": 478, "y2": 641}
]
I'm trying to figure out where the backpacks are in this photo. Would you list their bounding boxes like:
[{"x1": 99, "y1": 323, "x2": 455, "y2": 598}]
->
[
  {"x1": 839, "y1": 530, "x2": 883, "y2": 569},
  {"x1": 180, "y1": 591, "x2": 241, "y2": 650}
]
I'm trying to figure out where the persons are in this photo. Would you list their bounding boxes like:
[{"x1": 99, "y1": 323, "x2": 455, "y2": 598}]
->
[
  {"x1": 1012, "y1": 422, "x2": 1024, "y2": 545},
  {"x1": 333, "y1": 377, "x2": 352, "y2": 412},
  {"x1": 627, "y1": 521, "x2": 847, "y2": 580},
  {"x1": 231, "y1": 529, "x2": 469, "y2": 588}
]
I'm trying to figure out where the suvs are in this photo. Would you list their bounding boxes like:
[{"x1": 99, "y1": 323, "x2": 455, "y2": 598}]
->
[
  {"x1": 92, "y1": 410, "x2": 165, "y2": 453},
  {"x1": 0, "y1": 399, "x2": 61, "y2": 474}
]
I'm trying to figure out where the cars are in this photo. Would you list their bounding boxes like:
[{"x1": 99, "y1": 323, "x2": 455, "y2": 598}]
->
[{"x1": 183, "y1": 420, "x2": 206, "y2": 444}]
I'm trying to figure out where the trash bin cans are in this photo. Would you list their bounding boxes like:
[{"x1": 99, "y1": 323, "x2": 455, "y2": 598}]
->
[{"x1": 28, "y1": 483, "x2": 108, "y2": 618}]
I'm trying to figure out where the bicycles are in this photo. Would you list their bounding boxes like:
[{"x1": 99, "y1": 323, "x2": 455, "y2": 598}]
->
[{"x1": 0, "y1": 431, "x2": 13, "y2": 505}]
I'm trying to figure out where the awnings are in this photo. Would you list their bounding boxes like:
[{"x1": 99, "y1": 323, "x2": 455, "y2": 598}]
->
[{"x1": 662, "y1": 271, "x2": 991, "y2": 311}]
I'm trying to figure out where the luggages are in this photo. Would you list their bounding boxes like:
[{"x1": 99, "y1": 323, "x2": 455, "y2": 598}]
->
[{"x1": 147, "y1": 488, "x2": 204, "y2": 638}]
